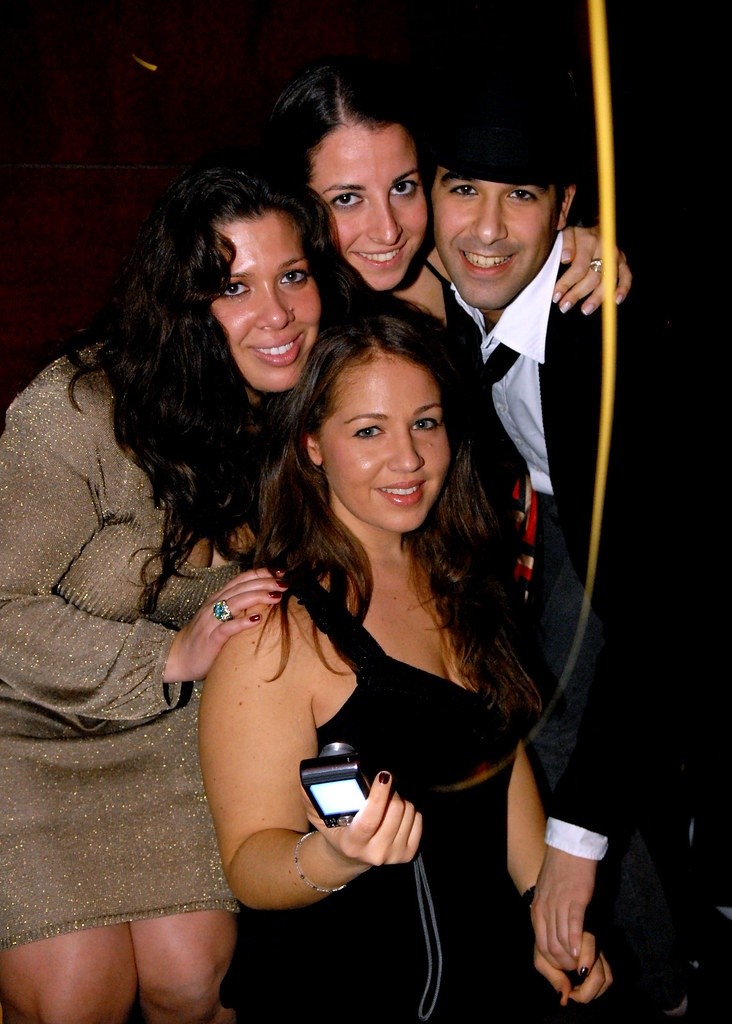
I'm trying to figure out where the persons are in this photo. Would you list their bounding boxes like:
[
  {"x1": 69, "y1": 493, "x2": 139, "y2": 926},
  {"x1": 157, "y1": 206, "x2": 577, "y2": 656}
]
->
[
  {"x1": 200, "y1": 297, "x2": 613, "y2": 1024},
  {"x1": 0, "y1": 165, "x2": 368, "y2": 1024},
  {"x1": 430, "y1": 82, "x2": 732, "y2": 1024},
  {"x1": 251, "y1": 59, "x2": 633, "y2": 362}
]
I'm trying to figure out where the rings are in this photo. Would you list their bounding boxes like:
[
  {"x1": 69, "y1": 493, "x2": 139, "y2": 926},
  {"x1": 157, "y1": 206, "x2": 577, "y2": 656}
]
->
[
  {"x1": 213, "y1": 601, "x2": 232, "y2": 622},
  {"x1": 591, "y1": 259, "x2": 602, "y2": 273}
]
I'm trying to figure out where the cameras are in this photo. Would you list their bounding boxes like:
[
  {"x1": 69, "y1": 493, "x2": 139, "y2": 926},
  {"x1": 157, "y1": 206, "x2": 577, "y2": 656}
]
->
[{"x1": 300, "y1": 741, "x2": 374, "y2": 828}]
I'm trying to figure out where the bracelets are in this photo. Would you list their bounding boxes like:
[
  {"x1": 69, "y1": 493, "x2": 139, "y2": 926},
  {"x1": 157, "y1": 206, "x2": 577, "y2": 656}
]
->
[
  {"x1": 294, "y1": 831, "x2": 346, "y2": 893},
  {"x1": 522, "y1": 885, "x2": 536, "y2": 906}
]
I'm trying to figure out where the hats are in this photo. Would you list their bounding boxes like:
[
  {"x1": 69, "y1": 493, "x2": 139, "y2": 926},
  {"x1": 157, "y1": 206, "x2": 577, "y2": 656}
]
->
[{"x1": 426, "y1": 75, "x2": 587, "y2": 188}]
